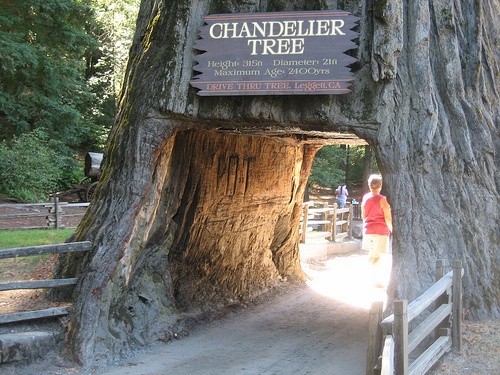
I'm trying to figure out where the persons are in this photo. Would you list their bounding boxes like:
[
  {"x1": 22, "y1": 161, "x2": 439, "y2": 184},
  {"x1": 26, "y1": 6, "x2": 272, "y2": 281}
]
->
[
  {"x1": 361, "y1": 174, "x2": 393, "y2": 288},
  {"x1": 334, "y1": 183, "x2": 349, "y2": 209}
]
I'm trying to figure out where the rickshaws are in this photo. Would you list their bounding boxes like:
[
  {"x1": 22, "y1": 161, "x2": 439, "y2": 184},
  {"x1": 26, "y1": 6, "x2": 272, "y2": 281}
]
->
[{"x1": 46, "y1": 151, "x2": 104, "y2": 202}]
